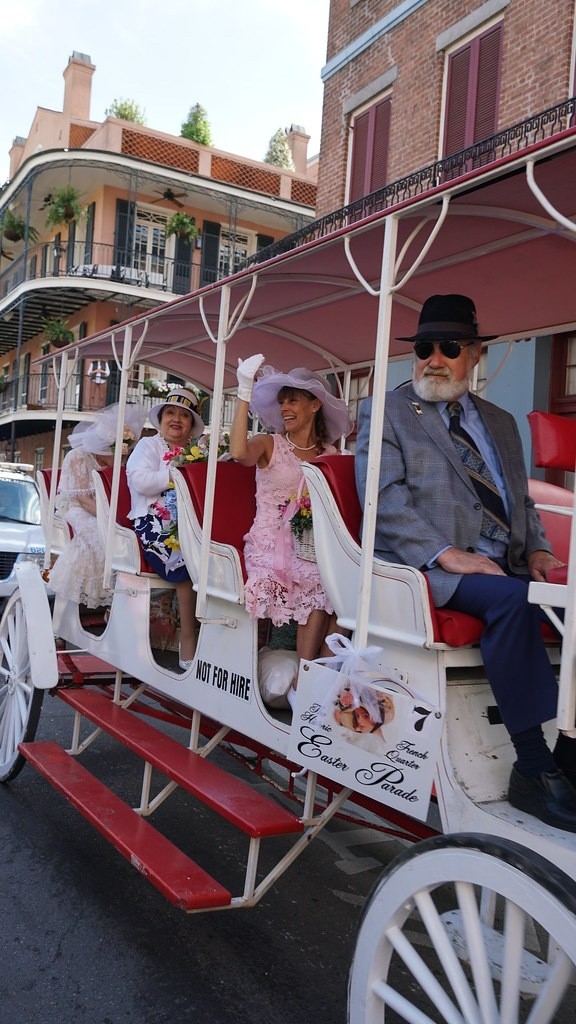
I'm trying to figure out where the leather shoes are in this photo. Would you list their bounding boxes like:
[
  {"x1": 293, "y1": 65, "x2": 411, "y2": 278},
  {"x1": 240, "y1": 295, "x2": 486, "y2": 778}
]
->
[
  {"x1": 508, "y1": 760, "x2": 576, "y2": 833},
  {"x1": 179, "y1": 640, "x2": 194, "y2": 670}
]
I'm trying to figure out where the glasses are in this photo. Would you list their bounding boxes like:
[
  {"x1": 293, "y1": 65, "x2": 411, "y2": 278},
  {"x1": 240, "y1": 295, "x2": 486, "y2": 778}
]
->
[{"x1": 413, "y1": 340, "x2": 476, "y2": 360}]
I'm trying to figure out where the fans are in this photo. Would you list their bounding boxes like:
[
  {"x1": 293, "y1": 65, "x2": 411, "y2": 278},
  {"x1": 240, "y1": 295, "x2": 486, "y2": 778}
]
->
[
  {"x1": 39, "y1": 192, "x2": 55, "y2": 211},
  {"x1": 0, "y1": 237, "x2": 14, "y2": 261},
  {"x1": 150, "y1": 187, "x2": 188, "y2": 207}
]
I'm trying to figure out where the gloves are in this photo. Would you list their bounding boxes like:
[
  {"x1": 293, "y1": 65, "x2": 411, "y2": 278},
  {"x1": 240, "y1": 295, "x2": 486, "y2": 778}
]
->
[{"x1": 236, "y1": 353, "x2": 265, "y2": 403}]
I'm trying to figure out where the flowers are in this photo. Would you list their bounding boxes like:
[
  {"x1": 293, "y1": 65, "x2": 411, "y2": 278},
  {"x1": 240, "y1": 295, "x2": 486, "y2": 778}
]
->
[
  {"x1": 279, "y1": 491, "x2": 314, "y2": 540},
  {"x1": 155, "y1": 481, "x2": 182, "y2": 551},
  {"x1": 163, "y1": 433, "x2": 231, "y2": 465},
  {"x1": 149, "y1": 380, "x2": 184, "y2": 392}
]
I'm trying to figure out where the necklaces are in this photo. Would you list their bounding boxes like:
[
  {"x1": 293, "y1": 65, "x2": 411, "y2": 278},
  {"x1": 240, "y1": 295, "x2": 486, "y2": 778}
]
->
[{"x1": 286, "y1": 433, "x2": 316, "y2": 450}]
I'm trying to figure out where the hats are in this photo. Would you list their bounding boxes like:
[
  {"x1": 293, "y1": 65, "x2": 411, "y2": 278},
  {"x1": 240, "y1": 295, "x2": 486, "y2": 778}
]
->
[
  {"x1": 395, "y1": 295, "x2": 499, "y2": 342},
  {"x1": 148, "y1": 389, "x2": 205, "y2": 439},
  {"x1": 249, "y1": 367, "x2": 355, "y2": 443},
  {"x1": 67, "y1": 401, "x2": 148, "y2": 454}
]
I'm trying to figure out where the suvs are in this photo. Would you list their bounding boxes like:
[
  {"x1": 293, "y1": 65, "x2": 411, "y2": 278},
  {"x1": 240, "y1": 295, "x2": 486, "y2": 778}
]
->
[{"x1": 0, "y1": 464, "x2": 59, "y2": 602}]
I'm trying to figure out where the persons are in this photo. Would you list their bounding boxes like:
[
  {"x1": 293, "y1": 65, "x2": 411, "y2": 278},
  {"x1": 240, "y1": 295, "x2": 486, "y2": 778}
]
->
[
  {"x1": 46, "y1": 402, "x2": 146, "y2": 623},
  {"x1": 335, "y1": 688, "x2": 394, "y2": 758},
  {"x1": 230, "y1": 353, "x2": 356, "y2": 715},
  {"x1": 354, "y1": 292, "x2": 576, "y2": 833},
  {"x1": 126, "y1": 388, "x2": 204, "y2": 670}
]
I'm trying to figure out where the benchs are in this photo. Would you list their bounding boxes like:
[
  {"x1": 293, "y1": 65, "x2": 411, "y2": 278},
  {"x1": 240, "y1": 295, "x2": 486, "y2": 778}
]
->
[
  {"x1": 168, "y1": 461, "x2": 263, "y2": 606},
  {"x1": 91, "y1": 466, "x2": 167, "y2": 590},
  {"x1": 301, "y1": 410, "x2": 575, "y2": 654},
  {"x1": 35, "y1": 469, "x2": 72, "y2": 560}
]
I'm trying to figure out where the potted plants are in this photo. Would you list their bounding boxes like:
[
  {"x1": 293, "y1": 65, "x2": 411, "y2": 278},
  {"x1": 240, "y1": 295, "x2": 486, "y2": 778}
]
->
[
  {"x1": 45, "y1": 186, "x2": 89, "y2": 227},
  {"x1": 0, "y1": 374, "x2": 6, "y2": 393},
  {"x1": 166, "y1": 212, "x2": 200, "y2": 242},
  {"x1": 42, "y1": 317, "x2": 72, "y2": 348},
  {"x1": 0, "y1": 208, "x2": 39, "y2": 243}
]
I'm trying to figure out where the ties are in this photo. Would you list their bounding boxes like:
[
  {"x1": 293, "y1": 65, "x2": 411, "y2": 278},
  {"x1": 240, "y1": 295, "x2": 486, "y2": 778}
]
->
[{"x1": 445, "y1": 400, "x2": 512, "y2": 544}]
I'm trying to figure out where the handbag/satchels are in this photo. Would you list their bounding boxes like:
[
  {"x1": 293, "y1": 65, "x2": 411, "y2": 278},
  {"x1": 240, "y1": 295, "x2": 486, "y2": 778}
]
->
[{"x1": 292, "y1": 454, "x2": 317, "y2": 563}]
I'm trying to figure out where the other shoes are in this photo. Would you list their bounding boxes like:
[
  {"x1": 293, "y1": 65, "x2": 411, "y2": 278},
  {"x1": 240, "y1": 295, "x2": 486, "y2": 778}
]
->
[
  {"x1": 103, "y1": 609, "x2": 111, "y2": 624},
  {"x1": 287, "y1": 685, "x2": 297, "y2": 710}
]
